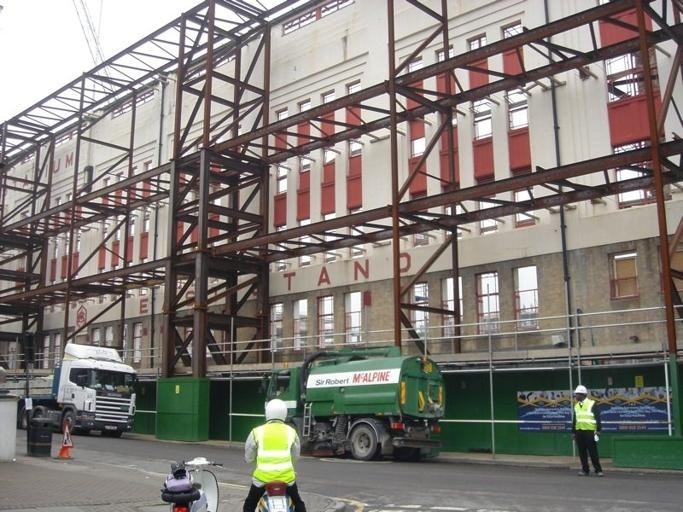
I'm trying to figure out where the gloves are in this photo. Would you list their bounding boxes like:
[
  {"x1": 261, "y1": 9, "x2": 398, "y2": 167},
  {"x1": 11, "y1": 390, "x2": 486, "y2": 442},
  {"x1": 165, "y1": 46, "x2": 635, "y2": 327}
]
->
[{"x1": 593, "y1": 431, "x2": 601, "y2": 438}]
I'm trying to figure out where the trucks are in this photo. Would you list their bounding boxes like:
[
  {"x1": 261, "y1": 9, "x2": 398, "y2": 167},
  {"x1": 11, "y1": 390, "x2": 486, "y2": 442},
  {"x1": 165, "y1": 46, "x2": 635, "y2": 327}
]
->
[
  {"x1": 0, "y1": 341, "x2": 143, "y2": 440},
  {"x1": 257, "y1": 343, "x2": 447, "y2": 463}
]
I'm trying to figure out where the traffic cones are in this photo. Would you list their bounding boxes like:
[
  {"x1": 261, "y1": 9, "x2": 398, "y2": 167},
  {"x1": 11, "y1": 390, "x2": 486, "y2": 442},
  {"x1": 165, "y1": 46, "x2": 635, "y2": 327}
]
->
[{"x1": 57, "y1": 421, "x2": 76, "y2": 459}]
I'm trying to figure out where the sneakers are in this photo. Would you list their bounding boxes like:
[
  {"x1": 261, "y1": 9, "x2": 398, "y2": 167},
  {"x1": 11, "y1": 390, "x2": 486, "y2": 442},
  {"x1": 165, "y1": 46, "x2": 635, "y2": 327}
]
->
[{"x1": 576, "y1": 470, "x2": 604, "y2": 476}]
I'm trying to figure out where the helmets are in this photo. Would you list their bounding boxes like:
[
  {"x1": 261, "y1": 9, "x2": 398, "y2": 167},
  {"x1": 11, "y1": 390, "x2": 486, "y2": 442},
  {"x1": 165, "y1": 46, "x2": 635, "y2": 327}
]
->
[
  {"x1": 572, "y1": 384, "x2": 588, "y2": 394},
  {"x1": 264, "y1": 397, "x2": 288, "y2": 422}
]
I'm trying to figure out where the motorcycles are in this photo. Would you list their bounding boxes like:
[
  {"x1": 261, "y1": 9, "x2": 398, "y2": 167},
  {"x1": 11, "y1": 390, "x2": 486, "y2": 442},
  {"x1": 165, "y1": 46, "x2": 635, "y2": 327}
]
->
[{"x1": 160, "y1": 455, "x2": 225, "y2": 512}]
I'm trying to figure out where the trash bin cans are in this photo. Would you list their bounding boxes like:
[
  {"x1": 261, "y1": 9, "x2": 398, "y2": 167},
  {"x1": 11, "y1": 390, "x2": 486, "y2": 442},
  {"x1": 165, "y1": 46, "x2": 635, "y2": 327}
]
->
[{"x1": 27, "y1": 418, "x2": 52, "y2": 456}]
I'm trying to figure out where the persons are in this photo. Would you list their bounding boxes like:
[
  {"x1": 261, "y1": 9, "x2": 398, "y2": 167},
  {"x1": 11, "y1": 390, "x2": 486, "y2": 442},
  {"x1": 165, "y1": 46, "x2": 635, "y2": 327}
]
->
[
  {"x1": 242, "y1": 397, "x2": 307, "y2": 512},
  {"x1": 570, "y1": 384, "x2": 604, "y2": 477}
]
